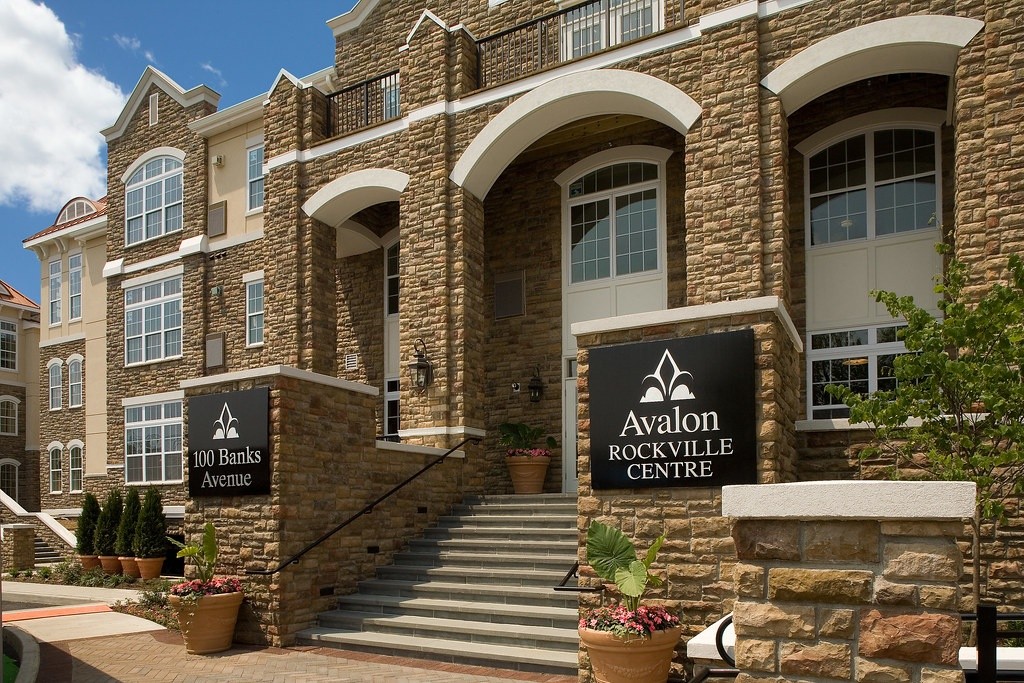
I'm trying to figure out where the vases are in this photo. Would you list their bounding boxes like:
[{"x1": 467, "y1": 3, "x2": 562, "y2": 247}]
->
[
  {"x1": 578, "y1": 623, "x2": 682, "y2": 683},
  {"x1": 169, "y1": 591, "x2": 242, "y2": 654},
  {"x1": 505, "y1": 456, "x2": 552, "y2": 494}
]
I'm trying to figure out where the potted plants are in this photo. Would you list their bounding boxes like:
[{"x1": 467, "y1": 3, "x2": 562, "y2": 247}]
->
[
  {"x1": 73, "y1": 488, "x2": 102, "y2": 570},
  {"x1": 134, "y1": 484, "x2": 166, "y2": 580},
  {"x1": 112, "y1": 484, "x2": 142, "y2": 578},
  {"x1": 91, "y1": 486, "x2": 125, "y2": 575}
]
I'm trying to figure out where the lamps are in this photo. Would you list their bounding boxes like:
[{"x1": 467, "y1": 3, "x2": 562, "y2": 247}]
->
[
  {"x1": 842, "y1": 357, "x2": 868, "y2": 366},
  {"x1": 407, "y1": 339, "x2": 433, "y2": 396},
  {"x1": 527, "y1": 366, "x2": 544, "y2": 402},
  {"x1": 841, "y1": 220, "x2": 853, "y2": 227}
]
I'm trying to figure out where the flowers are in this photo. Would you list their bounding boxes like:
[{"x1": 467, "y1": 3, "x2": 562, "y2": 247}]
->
[
  {"x1": 168, "y1": 578, "x2": 242, "y2": 636},
  {"x1": 504, "y1": 447, "x2": 552, "y2": 460},
  {"x1": 579, "y1": 604, "x2": 678, "y2": 645}
]
